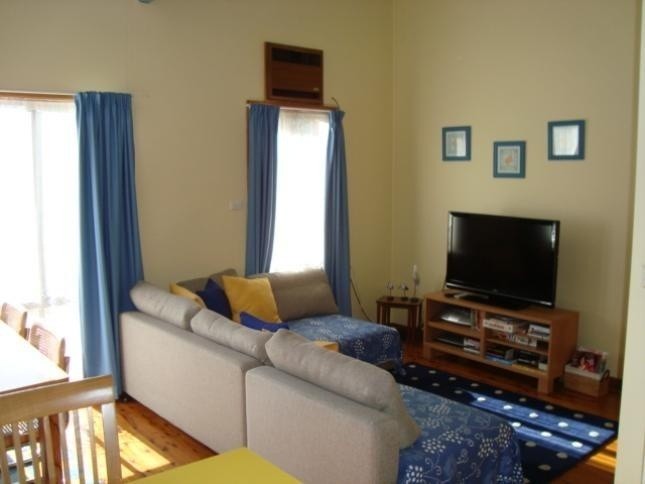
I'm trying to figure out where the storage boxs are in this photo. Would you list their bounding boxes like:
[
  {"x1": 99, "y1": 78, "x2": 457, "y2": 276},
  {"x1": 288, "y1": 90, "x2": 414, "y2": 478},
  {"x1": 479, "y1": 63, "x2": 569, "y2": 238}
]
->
[{"x1": 559, "y1": 370, "x2": 608, "y2": 397}]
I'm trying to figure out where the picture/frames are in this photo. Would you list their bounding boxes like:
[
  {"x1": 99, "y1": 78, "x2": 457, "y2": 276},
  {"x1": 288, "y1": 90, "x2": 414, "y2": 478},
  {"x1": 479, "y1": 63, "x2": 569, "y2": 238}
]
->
[
  {"x1": 441, "y1": 126, "x2": 472, "y2": 160},
  {"x1": 545, "y1": 120, "x2": 584, "y2": 159},
  {"x1": 492, "y1": 141, "x2": 526, "y2": 178}
]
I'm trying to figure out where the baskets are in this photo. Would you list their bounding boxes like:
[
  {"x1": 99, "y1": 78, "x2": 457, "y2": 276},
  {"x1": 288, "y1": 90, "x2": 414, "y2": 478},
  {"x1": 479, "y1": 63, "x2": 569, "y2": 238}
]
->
[{"x1": 561, "y1": 362, "x2": 611, "y2": 398}]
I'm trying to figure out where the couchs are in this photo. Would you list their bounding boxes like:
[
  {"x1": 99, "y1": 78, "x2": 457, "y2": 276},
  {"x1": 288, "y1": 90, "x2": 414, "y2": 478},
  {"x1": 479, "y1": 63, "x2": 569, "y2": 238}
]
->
[{"x1": 118, "y1": 271, "x2": 529, "y2": 484}]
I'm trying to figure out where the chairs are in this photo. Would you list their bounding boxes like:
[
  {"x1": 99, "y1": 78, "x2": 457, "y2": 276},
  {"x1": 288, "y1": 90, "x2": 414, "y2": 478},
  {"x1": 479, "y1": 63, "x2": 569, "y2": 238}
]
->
[
  {"x1": 0, "y1": 302, "x2": 30, "y2": 340},
  {"x1": 0, "y1": 374, "x2": 122, "y2": 484},
  {"x1": 0, "y1": 321, "x2": 69, "y2": 472}
]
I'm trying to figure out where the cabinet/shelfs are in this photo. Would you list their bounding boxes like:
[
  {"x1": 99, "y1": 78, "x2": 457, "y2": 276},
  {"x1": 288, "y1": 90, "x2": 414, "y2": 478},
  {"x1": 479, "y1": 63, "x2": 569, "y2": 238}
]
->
[{"x1": 419, "y1": 289, "x2": 577, "y2": 397}]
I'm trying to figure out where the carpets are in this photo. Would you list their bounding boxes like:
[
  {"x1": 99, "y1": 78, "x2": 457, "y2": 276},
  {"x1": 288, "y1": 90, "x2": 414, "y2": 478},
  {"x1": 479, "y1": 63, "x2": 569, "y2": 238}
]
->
[{"x1": 388, "y1": 361, "x2": 617, "y2": 484}]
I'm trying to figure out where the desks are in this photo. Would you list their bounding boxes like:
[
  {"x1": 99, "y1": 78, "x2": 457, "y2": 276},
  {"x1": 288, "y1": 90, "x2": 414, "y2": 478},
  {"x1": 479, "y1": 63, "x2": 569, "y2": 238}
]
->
[
  {"x1": 128, "y1": 447, "x2": 301, "y2": 483},
  {"x1": 0, "y1": 319, "x2": 69, "y2": 392},
  {"x1": 377, "y1": 296, "x2": 423, "y2": 344}
]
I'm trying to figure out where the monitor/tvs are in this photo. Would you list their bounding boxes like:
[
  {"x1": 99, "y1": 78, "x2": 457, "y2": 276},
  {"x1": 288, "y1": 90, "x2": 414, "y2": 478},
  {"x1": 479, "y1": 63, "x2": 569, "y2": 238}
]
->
[{"x1": 445, "y1": 210, "x2": 560, "y2": 311}]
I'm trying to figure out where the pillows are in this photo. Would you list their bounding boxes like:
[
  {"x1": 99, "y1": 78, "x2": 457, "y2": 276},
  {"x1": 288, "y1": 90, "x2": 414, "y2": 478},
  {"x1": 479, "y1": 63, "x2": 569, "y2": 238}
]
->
[
  {"x1": 246, "y1": 268, "x2": 339, "y2": 320},
  {"x1": 195, "y1": 277, "x2": 233, "y2": 320},
  {"x1": 311, "y1": 339, "x2": 339, "y2": 352},
  {"x1": 175, "y1": 268, "x2": 238, "y2": 294},
  {"x1": 264, "y1": 329, "x2": 421, "y2": 450},
  {"x1": 240, "y1": 311, "x2": 290, "y2": 334},
  {"x1": 222, "y1": 275, "x2": 282, "y2": 323},
  {"x1": 169, "y1": 282, "x2": 207, "y2": 308},
  {"x1": 130, "y1": 280, "x2": 200, "y2": 330},
  {"x1": 189, "y1": 308, "x2": 272, "y2": 366}
]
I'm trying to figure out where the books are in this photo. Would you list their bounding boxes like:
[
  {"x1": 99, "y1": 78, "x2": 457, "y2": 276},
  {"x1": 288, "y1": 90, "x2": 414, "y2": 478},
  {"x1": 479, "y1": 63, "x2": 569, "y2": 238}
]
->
[
  {"x1": 483, "y1": 313, "x2": 551, "y2": 376},
  {"x1": 565, "y1": 346, "x2": 608, "y2": 381}
]
